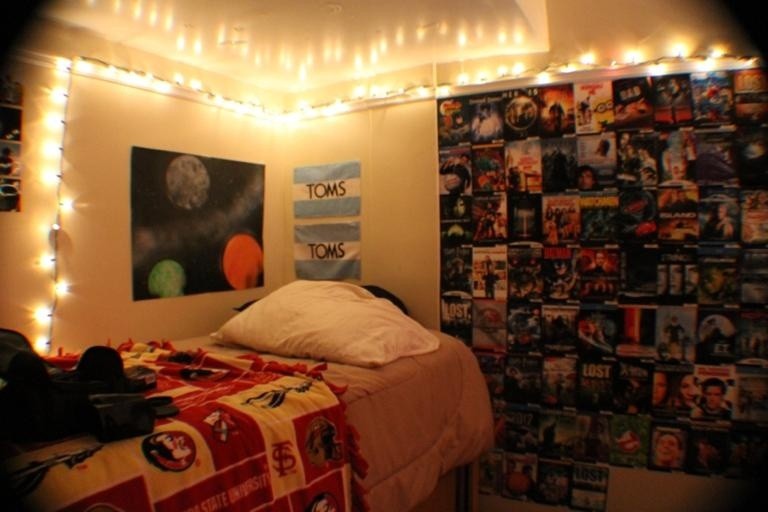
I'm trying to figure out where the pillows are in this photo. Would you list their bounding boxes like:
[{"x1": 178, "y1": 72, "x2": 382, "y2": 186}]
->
[{"x1": 209, "y1": 280, "x2": 440, "y2": 365}]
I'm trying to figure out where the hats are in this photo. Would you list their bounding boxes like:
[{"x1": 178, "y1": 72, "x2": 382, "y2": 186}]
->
[{"x1": 50, "y1": 346, "x2": 156, "y2": 393}]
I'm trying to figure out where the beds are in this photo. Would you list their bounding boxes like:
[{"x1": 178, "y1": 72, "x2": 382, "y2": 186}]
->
[{"x1": 0, "y1": 284, "x2": 495, "y2": 512}]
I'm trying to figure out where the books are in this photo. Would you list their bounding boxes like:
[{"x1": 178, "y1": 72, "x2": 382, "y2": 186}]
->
[{"x1": 437, "y1": 75, "x2": 767, "y2": 512}]
